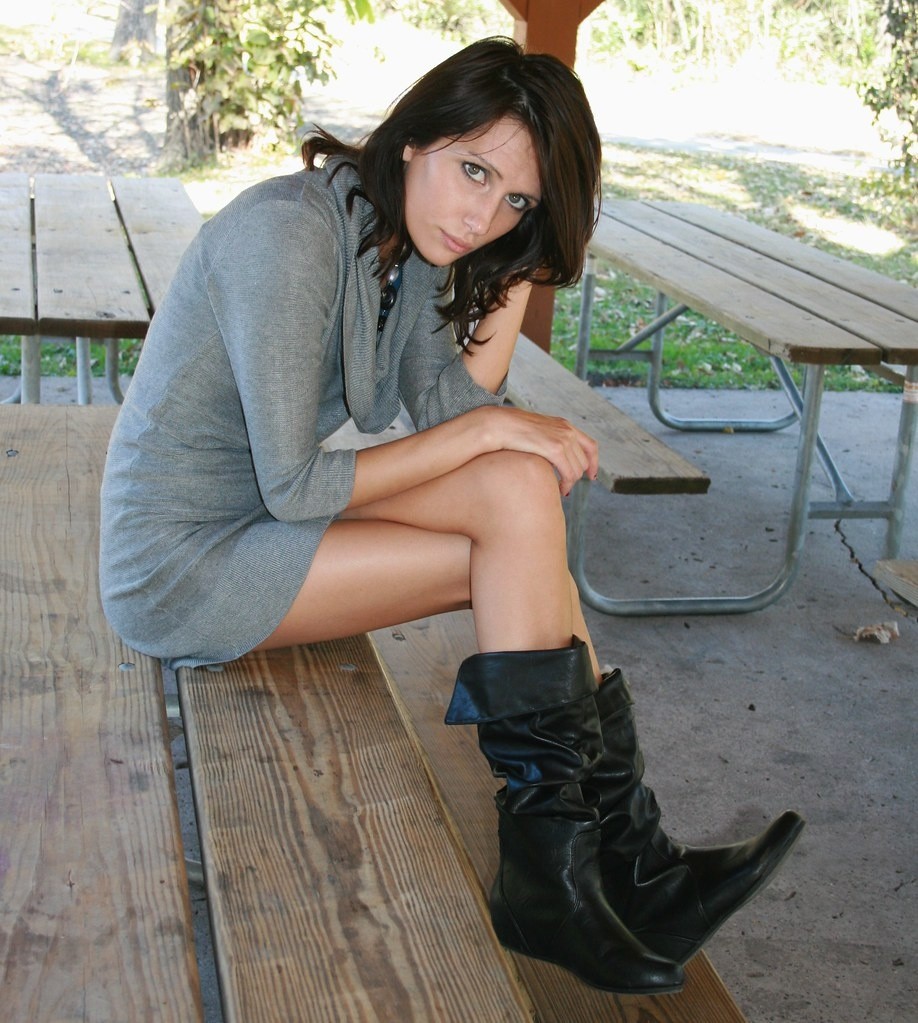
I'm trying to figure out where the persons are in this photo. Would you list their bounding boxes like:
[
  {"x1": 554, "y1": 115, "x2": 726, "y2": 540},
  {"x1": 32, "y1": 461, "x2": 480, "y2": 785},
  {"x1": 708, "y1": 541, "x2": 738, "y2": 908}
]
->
[{"x1": 99, "y1": 36, "x2": 804, "y2": 998}]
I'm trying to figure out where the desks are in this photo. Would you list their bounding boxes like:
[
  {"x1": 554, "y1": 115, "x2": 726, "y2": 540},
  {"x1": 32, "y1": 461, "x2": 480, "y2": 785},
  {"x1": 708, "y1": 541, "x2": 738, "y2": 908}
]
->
[
  {"x1": 0, "y1": 404, "x2": 533, "y2": 1023},
  {"x1": 0, "y1": 173, "x2": 205, "y2": 409},
  {"x1": 587, "y1": 198, "x2": 918, "y2": 605}
]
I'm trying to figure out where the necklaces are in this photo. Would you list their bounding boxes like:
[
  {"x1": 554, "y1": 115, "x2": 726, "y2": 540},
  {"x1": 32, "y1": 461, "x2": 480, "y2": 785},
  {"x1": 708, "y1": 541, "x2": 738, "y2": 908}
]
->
[{"x1": 377, "y1": 241, "x2": 409, "y2": 332}]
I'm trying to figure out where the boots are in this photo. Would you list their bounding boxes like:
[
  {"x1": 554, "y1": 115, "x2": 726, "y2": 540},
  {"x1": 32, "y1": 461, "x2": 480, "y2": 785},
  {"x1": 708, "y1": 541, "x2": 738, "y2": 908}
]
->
[
  {"x1": 581, "y1": 667, "x2": 807, "y2": 966},
  {"x1": 444, "y1": 632, "x2": 686, "y2": 995}
]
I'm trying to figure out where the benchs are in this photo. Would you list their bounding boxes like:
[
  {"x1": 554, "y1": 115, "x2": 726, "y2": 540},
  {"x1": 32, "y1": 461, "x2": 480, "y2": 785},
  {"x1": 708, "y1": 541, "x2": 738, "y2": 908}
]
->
[
  {"x1": 464, "y1": 321, "x2": 712, "y2": 497},
  {"x1": 364, "y1": 607, "x2": 750, "y2": 1023}
]
